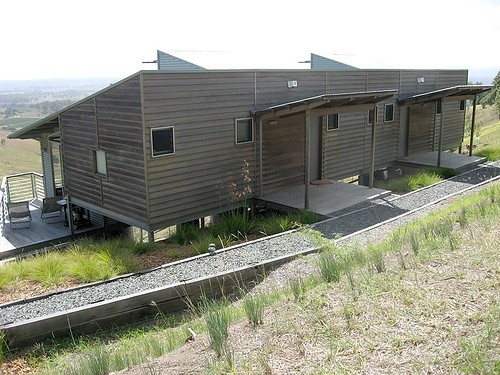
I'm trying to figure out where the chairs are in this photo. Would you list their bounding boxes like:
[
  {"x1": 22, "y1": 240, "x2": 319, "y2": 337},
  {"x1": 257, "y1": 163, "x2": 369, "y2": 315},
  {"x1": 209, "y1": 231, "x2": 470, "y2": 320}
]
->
[
  {"x1": 39, "y1": 195, "x2": 64, "y2": 225},
  {"x1": 6, "y1": 200, "x2": 33, "y2": 230}
]
